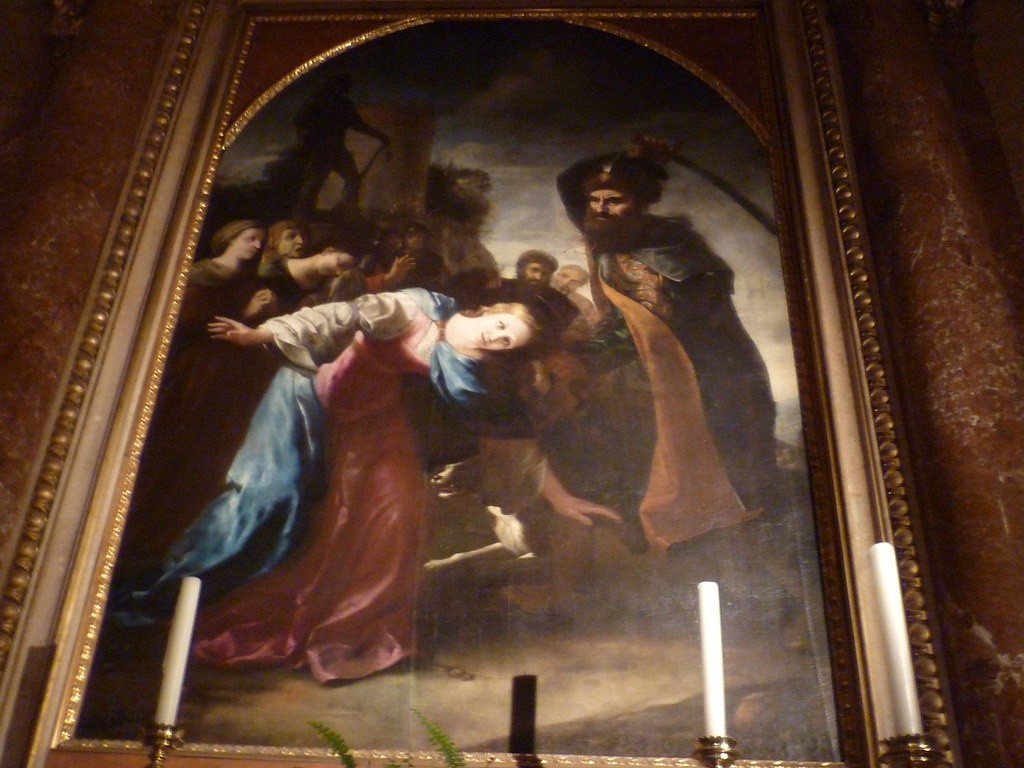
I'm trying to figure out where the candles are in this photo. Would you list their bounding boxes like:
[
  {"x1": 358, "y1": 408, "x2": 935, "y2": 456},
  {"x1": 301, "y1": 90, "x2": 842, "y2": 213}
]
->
[
  {"x1": 696, "y1": 581, "x2": 726, "y2": 737},
  {"x1": 869, "y1": 542, "x2": 922, "y2": 737},
  {"x1": 155, "y1": 576, "x2": 201, "y2": 725}
]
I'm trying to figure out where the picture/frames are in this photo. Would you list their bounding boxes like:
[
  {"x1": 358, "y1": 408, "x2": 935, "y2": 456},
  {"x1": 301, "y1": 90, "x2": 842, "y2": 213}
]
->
[{"x1": 2, "y1": 1, "x2": 959, "y2": 768}]
